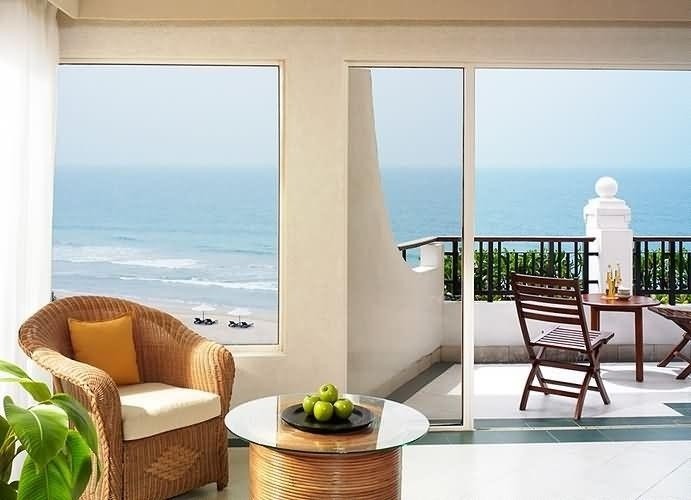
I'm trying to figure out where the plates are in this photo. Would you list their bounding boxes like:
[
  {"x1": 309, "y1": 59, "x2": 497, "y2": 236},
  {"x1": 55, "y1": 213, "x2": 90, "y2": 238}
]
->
[{"x1": 280, "y1": 401, "x2": 376, "y2": 434}]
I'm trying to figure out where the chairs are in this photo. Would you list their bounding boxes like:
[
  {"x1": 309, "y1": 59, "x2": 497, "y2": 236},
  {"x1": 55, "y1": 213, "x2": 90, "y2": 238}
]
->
[
  {"x1": 647, "y1": 304, "x2": 690, "y2": 379},
  {"x1": 15, "y1": 295, "x2": 235, "y2": 500},
  {"x1": 511, "y1": 270, "x2": 614, "y2": 420}
]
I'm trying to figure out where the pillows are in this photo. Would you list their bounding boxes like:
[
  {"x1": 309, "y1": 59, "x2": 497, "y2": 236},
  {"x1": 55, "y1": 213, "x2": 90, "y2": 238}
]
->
[{"x1": 68, "y1": 312, "x2": 141, "y2": 387}]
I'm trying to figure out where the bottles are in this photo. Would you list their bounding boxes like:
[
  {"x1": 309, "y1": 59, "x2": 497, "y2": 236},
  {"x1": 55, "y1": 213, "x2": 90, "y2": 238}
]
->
[{"x1": 606, "y1": 264, "x2": 621, "y2": 297}]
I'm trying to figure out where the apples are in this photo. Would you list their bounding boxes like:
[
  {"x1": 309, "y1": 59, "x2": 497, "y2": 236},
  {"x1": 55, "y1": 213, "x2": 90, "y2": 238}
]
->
[
  {"x1": 334, "y1": 399, "x2": 353, "y2": 419},
  {"x1": 314, "y1": 401, "x2": 333, "y2": 422},
  {"x1": 303, "y1": 395, "x2": 320, "y2": 416},
  {"x1": 320, "y1": 384, "x2": 338, "y2": 404}
]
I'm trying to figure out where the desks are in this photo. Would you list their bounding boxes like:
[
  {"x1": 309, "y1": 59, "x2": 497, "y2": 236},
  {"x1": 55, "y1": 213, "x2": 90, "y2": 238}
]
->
[{"x1": 577, "y1": 293, "x2": 661, "y2": 384}]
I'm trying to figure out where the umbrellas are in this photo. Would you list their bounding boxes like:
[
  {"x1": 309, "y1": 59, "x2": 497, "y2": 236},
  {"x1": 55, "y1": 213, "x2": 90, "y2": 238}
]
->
[
  {"x1": 192, "y1": 303, "x2": 217, "y2": 321},
  {"x1": 227, "y1": 307, "x2": 253, "y2": 325}
]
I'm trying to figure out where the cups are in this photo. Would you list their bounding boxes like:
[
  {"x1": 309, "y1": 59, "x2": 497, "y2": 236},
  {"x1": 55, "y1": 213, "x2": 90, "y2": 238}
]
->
[{"x1": 616, "y1": 288, "x2": 632, "y2": 301}]
